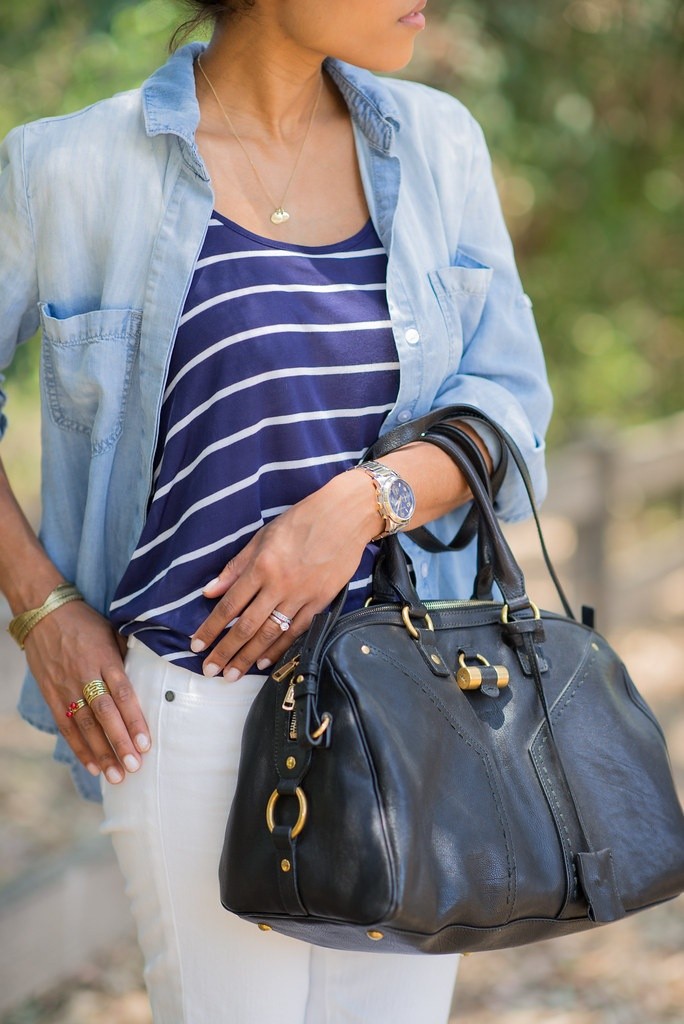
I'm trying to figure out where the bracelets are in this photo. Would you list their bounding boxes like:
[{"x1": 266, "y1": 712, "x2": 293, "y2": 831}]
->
[{"x1": 6, "y1": 582, "x2": 86, "y2": 650}]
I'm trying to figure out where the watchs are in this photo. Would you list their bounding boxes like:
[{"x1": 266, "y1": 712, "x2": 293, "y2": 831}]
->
[{"x1": 348, "y1": 461, "x2": 416, "y2": 542}]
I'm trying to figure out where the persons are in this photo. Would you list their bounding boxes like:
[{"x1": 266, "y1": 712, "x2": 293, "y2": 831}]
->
[{"x1": 0, "y1": 0, "x2": 554, "y2": 1024}]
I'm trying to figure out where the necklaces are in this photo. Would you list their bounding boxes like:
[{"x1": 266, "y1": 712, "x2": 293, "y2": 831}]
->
[{"x1": 196, "y1": 52, "x2": 324, "y2": 224}]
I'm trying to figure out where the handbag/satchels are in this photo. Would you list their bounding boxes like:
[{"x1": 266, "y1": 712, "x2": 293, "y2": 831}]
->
[{"x1": 218, "y1": 405, "x2": 684, "y2": 953}]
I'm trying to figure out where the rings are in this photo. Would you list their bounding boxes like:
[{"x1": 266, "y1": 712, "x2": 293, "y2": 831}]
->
[
  {"x1": 66, "y1": 699, "x2": 87, "y2": 717},
  {"x1": 269, "y1": 610, "x2": 293, "y2": 631},
  {"x1": 83, "y1": 681, "x2": 110, "y2": 706}
]
